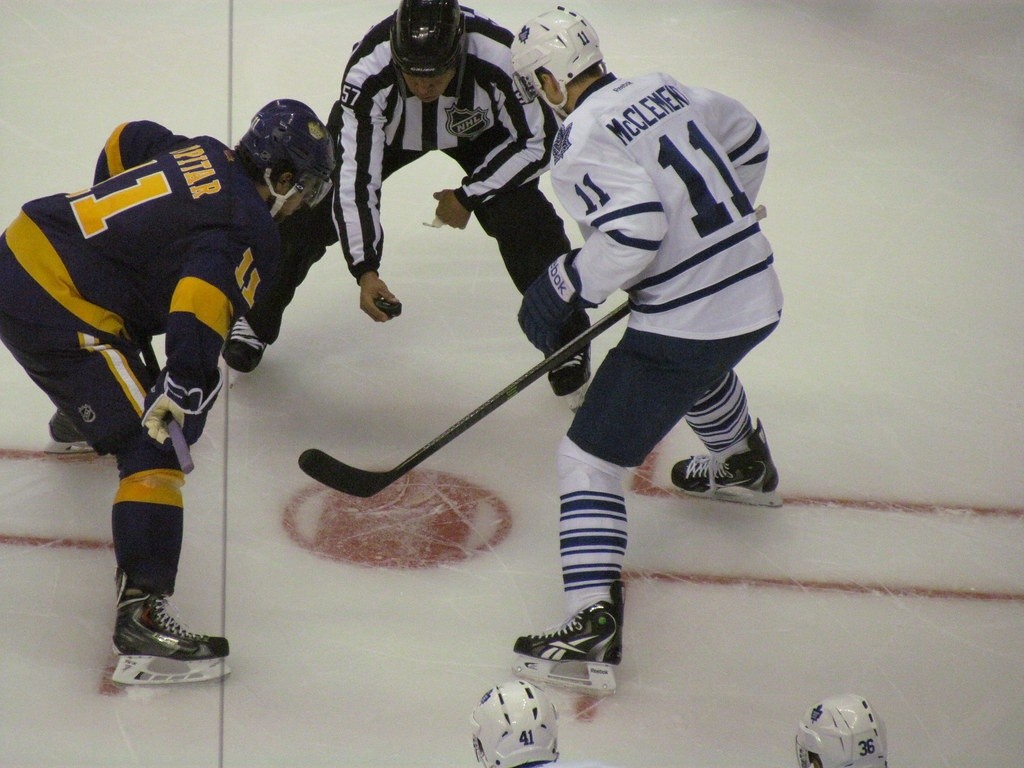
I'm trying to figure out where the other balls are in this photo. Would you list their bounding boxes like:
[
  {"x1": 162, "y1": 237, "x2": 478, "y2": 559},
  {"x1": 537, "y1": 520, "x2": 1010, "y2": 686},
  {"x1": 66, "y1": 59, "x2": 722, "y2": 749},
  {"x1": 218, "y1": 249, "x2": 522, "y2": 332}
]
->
[{"x1": 375, "y1": 299, "x2": 403, "y2": 317}]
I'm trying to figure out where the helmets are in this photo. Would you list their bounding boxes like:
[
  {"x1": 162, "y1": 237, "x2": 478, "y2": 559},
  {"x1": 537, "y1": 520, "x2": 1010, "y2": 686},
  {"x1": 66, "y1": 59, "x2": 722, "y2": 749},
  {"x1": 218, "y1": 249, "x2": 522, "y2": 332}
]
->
[
  {"x1": 389, "y1": 0, "x2": 463, "y2": 79},
  {"x1": 239, "y1": 98, "x2": 336, "y2": 183},
  {"x1": 511, "y1": 6, "x2": 603, "y2": 92},
  {"x1": 795, "y1": 695, "x2": 886, "y2": 768},
  {"x1": 470, "y1": 678, "x2": 560, "y2": 768}
]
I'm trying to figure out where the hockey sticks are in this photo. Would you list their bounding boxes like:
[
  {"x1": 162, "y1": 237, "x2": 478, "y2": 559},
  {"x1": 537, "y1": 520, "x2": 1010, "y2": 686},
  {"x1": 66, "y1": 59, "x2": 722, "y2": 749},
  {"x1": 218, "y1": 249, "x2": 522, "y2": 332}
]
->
[
  {"x1": 296, "y1": 202, "x2": 769, "y2": 501},
  {"x1": 139, "y1": 335, "x2": 198, "y2": 475}
]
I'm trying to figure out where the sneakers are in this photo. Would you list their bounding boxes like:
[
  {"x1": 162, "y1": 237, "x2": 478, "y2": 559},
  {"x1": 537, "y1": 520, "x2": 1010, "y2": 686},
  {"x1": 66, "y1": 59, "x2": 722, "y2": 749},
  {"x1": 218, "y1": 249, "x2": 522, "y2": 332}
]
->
[
  {"x1": 512, "y1": 581, "x2": 625, "y2": 697},
  {"x1": 672, "y1": 420, "x2": 784, "y2": 508},
  {"x1": 45, "y1": 401, "x2": 98, "y2": 454},
  {"x1": 546, "y1": 314, "x2": 591, "y2": 411},
  {"x1": 112, "y1": 565, "x2": 233, "y2": 687},
  {"x1": 220, "y1": 315, "x2": 268, "y2": 388}
]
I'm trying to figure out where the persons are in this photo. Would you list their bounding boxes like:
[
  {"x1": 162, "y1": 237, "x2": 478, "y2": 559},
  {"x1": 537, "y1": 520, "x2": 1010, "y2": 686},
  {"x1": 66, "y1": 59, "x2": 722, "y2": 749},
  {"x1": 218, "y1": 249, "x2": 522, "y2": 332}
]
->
[
  {"x1": 508, "y1": 7, "x2": 784, "y2": 700},
  {"x1": 1, "y1": 98, "x2": 339, "y2": 686},
  {"x1": 220, "y1": 0, "x2": 594, "y2": 413},
  {"x1": 472, "y1": 679, "x2": 621, "y2": 768},
  {"x1": 794, "y1": 691, "x2": 887, "y2": 768}
]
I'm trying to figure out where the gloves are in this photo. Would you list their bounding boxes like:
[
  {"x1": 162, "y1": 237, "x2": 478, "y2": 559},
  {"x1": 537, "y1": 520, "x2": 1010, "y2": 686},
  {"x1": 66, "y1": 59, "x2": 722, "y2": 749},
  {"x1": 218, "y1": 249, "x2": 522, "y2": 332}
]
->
[
  {"x1": 516, "y1": 250, "x2": 603, "y2": 357},
  {"x1": 139, "y1": 365, "x2": 224, "y2": 453}
]
